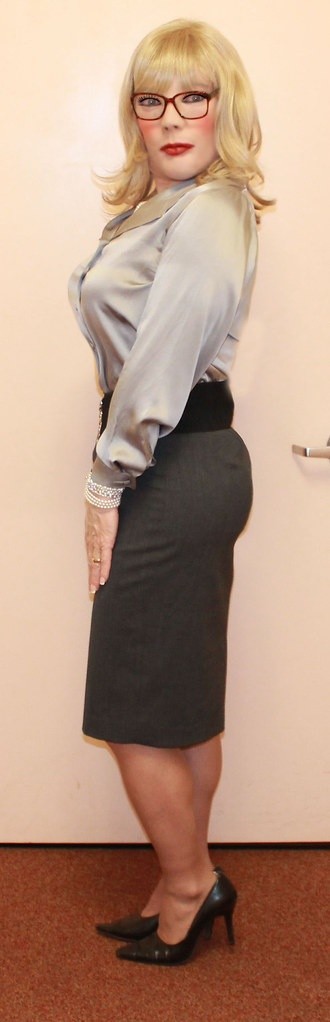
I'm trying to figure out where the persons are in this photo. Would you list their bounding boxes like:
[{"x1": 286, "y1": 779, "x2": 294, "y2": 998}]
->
[{"x1": 66, "y1": 18, "x2": 275, "y2": 967}]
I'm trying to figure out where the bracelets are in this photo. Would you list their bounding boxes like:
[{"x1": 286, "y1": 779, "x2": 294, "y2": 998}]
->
[{"x1": 81, "y1": 474, "x2": 125, "y2": 510}]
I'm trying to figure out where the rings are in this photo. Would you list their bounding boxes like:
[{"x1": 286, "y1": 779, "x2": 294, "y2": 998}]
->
[{"x1": 92, "y1": 558, "x2": 100, "y2": 563}]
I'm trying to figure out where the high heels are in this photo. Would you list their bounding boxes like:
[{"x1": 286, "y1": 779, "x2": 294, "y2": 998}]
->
[
  {"x1": 96, "y1": 866, "x2": 221, "y2": 942},
  {"x1": 116, "y1": 873, "x2": 238, "y2": 966}
]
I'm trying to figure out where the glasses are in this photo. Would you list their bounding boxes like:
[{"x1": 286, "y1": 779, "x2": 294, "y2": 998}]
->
[{"x1": 131, "y1": 89, "x2": 218, "y2": 120}]
